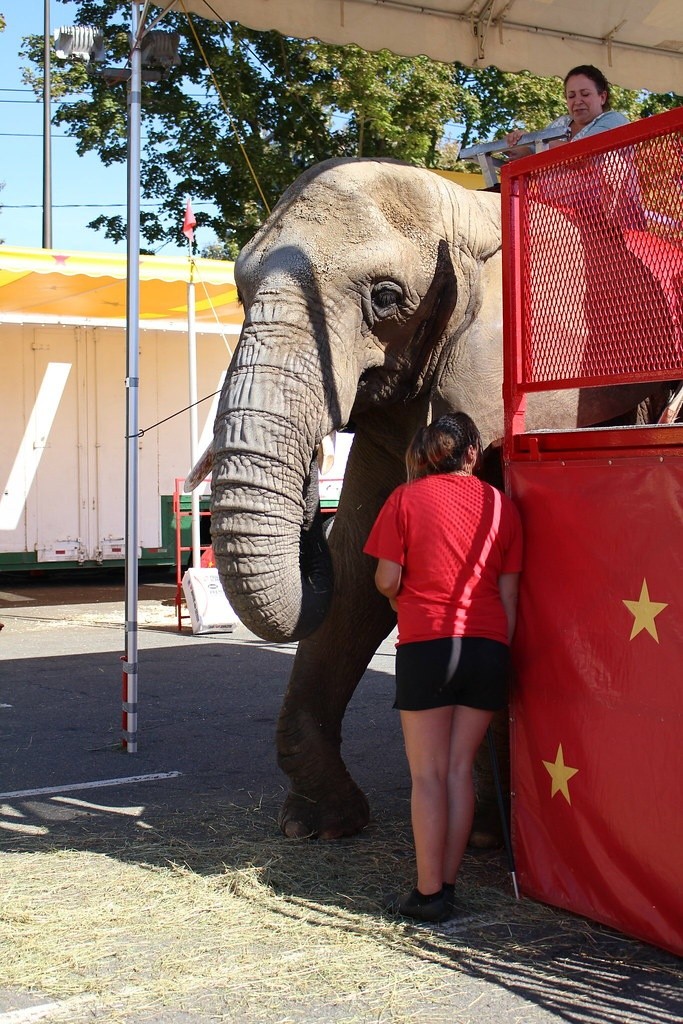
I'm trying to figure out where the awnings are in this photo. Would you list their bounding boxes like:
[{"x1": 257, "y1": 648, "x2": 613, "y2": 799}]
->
[{"x1": 0, "y1": 245, "x2": 243, "y2": 324}]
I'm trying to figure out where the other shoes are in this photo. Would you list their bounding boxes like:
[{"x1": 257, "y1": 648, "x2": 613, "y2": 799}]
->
[{"x1": 382, "y1": 892, "x2": 454, "y2": 922}]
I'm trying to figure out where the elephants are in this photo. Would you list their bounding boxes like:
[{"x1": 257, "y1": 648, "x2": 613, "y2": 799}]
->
[{"x1": 213, "y1": 157, "x2": 683, "y2": 853}]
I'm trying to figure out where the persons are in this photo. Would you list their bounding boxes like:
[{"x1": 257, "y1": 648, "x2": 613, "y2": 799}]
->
[
  {"x1": 362, "y1": 411, "x2": 524, "y2": 923},
  {"x1": 480, "y1": 65, "x2": 648, "y2": 238}
]
